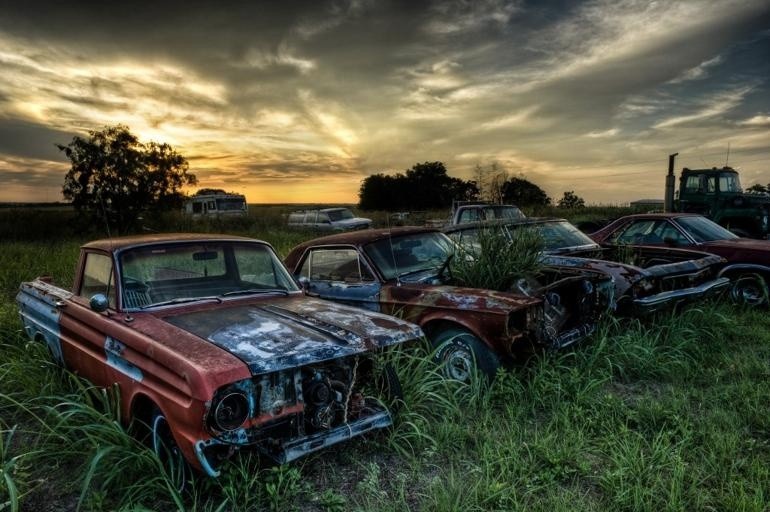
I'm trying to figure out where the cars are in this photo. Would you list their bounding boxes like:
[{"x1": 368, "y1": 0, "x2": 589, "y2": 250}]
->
[
  {"x1": 453, "y1": 205, "x2": 527, "y2": 226},
  {"x1": 288, "y1": 208, "x2": 373, "y2": 233}
]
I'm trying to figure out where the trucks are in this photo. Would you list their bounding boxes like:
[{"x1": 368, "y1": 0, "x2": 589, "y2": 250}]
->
[
  {"x1": 665, "y1": 153, "x2": 770, "y2": 232},
  {"x1": 185, "y1": 195, "x2": 248, "y2": 217}
]
